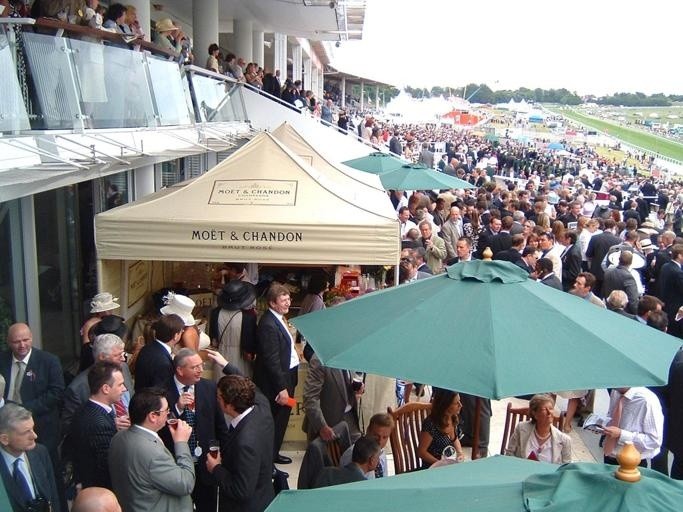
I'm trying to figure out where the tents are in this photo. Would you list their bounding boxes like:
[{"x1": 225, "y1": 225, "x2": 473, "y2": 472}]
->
[
  {"x1": 92, "y1": 129, "x2": 401, "y2": 326},
  {"x1": 171, "y1": 120, "x2": 398, "y2": 219}
]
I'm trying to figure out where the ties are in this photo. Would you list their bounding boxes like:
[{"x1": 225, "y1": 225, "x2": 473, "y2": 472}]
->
[
  {"x1": 408, "y1": 279, "x2": 413, "y2": 283},
  {"x1": 282, "y1": 316, "x2": 296, "y2": 350},
  {"x1": 375, "y1": 462, "x2": 383, "y2": 479},
  {"x1": 13, "y1": 362, "x2": 25, "y2": 403},
  {"x1": 228, "y1": 423, "x2": 235, "y2": 435},
  {"x1": 12, "y1": 459, "x2": 34, "y2": 504},
  {"x1": 342, "y1": 369, "x2": 353, "y2": 407},
  {"x1": 183, "y1": 385, "x2": 196, "y2": 456},
  {"x1": 603, "y1": 396, "x2": 624, "y2": 457},
  {"x1": 114, "y1": 400, "x2": 127, "y2": 418},
  {"x1": 110, "y1": 409, "x2": 114, "y2": 418}
]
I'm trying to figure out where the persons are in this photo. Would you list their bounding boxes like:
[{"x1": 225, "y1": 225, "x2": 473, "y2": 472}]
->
[
  {"x1": 245, "y1": 54, "x2": 403, "y2": 173},
  {"x1": 297, "y1": 272, "x2": 330, "y2": 317},
  {"x1": 134, "y1": 314, "x2": 184, "y2": 393},
  {"x1": 67, "y1": 333, "x2": 134, "y2": 431},
  {"x1": 0, "y1": 0, "x2": 143, "y2": 135},
  {"x1": 549, "y1": 389, "x2": 588, "y2": 432},
  {"x1": 158, "y1": 348, "x2": 229, "y2": 511},
  {"x1": 0, "y1": 322, "x2": 66, "y2": 467},
  {"x1": 209, "y1": 280, "x2": 258, "y2": 430},
  {"x1": 0, "y1": 404, "x2": 61, "y2": 511},
  {"x1": 417, "y1": 388, "x2": 464, "y2": 469},
  {"x1": 310, "y1": 440, "x2": 382, "y2": 491},
  {"x1": 233, "y1": 57, "x2": 246, "y2": 82},
  {"x1": 254, "y1": 284, "x2": 300, "y2": 465},
  {"x1": 458, "y1": 392, "x2": 492, "y2": 458},
  {"x1": 339, "y1": 413, "x2": 394, "y2": 480},
  {"x1": 168, "y1": 20, "x2": 194, "y2": 65},
  {"x1": 228, "y1": 263, "x2": 251, "y2": 284},
  {"x1": 506, "y1": 394, "x2": 572, "y2": 464},
  {"x1": 400, "y1": 186, "x2": 605, "y2": 308},
  {"x1": 80, "y1": 291, "x2": 121, "y2": 344},
  {"x1": 68, "y1": 360, "x2": 127, "y2": 489},
  {"x1": 150, "y1": 18, "x2": 185, "y2": 61},
  {"x1": 301, "y1": 352, "x2": 365, "y2": 454},
  {"x1": 71, "y1": 487, "x2": 122, "y2": 512},
  {"x1": 604, "y1": 187, "x2": 683, "y2": 339},
  {"x1": 206, "y1": 43, "x2": 220, "y2": 78},
  {"x1": 594, "y1": 386, "x2": 664, "y2": 469},
  {"x1": 78, "y1": 316, "x2": 129, "y2": 374},
  {"x1": 106, "y1": 387, "x2": 196, "y2": 512},
  {"x1": 160, "y1": 291, "x2": 200, "y2": 353},
  {"x1": 222, "y1": 53, "x2": 238, "y2": 78},
  {"x1": 200, "y1": 348, "x2": 275, "y2": 512}
]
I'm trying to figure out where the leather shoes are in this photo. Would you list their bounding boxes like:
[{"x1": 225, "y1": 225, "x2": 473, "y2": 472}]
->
[
  {"x1": 273, "y1": 455, "x2": 292, "y2": 464},
  {"x1": 271, "y1": 469, "x2": 289, "y2": 479},
  {"x1": 478, "y1": 447, "x2": 488, "y2": 458},
  {"x1": 458, "y1": 434, "x2": 474, "y2": 447}
]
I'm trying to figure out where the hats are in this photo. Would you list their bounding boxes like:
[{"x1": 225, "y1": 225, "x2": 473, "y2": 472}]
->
[
  {"x1": 607, "y1": 243, "x2": 645, "y2": 269},
  {"x1": 217, "y1": 281, "x2": 256, "y2": 312},
  {"x1": 89, "y1": 293, "x2": 121, "y2": 313},
  {"x1": 546, "y1": 180, "x2": 559, "y2": 188},
  {"x1": 88, "y1": 314, "x2": 127, "y2": 345},
  {"x1": 154, "y1": 18, "x2": 179, "y2": 31},
  {"x1": 161, "y1": 291, "x2": 196, "y2": 327}
]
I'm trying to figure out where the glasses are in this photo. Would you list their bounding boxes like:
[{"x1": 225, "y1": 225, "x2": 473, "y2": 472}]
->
[
  {"x1": 155, "y1": 408, "x2": 170, "y2": 415},
  {"x1": 103, "y1": 351, "x2": 125, "y2": 358},
  {"x1": 191, "y1": 362, "x2": 205, "y2": 372}
]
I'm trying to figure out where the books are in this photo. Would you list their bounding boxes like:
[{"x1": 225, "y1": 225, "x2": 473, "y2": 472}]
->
[{"x1": 582, "y1": 413, "x2": 612, "y2": 433}]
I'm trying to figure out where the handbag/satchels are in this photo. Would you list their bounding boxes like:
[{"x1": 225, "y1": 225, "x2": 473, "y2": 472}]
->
[{"x1": 441, "y1": 446, "x2": 457, "y2": 461}]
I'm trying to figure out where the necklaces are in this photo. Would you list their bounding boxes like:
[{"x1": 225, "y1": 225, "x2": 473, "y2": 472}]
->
[{"x1": 534, "y1": 428, "x2": 551, "y2": 440}]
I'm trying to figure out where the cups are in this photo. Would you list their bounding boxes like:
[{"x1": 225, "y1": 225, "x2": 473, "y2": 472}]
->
[
  {"x1": 167, "y1": 419, "x2": 178, "y2": 431},
  {"x1": 209, "y1": 447, "x2": 217, "y2": 460},
  {"x1": 188, "y1": 396, "x2": 194, "y2": 410}
]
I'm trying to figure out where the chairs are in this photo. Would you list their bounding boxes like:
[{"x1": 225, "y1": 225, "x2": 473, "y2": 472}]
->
[
  {"x1": 312, "y1": 421, "x2": 352, "y2": 468},
  {"x1": 387, "y1": 403, "x2": 437, "y2": 474},
  {"x1": 501, "y1": 402, "x2": 565, "y2": 456}
]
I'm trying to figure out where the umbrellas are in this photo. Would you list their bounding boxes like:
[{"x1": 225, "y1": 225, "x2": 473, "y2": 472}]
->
[
  {"x1": 262, "y1": 441, "x2": 683, "y2": 512},
  {"x1": 289, "y1": 246, "x2": 683, "y2": 463}
]
